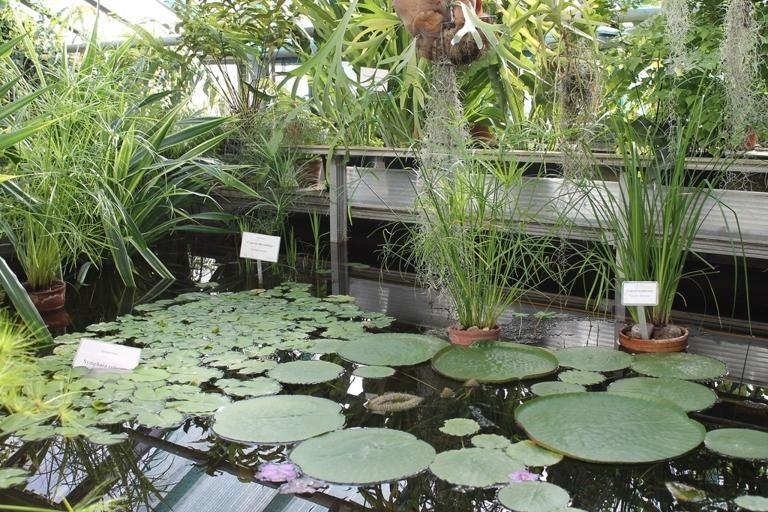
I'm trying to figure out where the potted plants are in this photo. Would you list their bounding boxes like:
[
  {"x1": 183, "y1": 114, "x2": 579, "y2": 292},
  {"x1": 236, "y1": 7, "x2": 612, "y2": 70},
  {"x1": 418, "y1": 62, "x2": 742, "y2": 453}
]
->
[
  {"x1": 567, "y1": 68, "x2": 765, "y2": 355},
  {"x1": 363, "y1": 85, "x2": 564, "y2": 344},
  {"x1": 1, "y1": 36, "x2": 178, "y2": 314}
]
